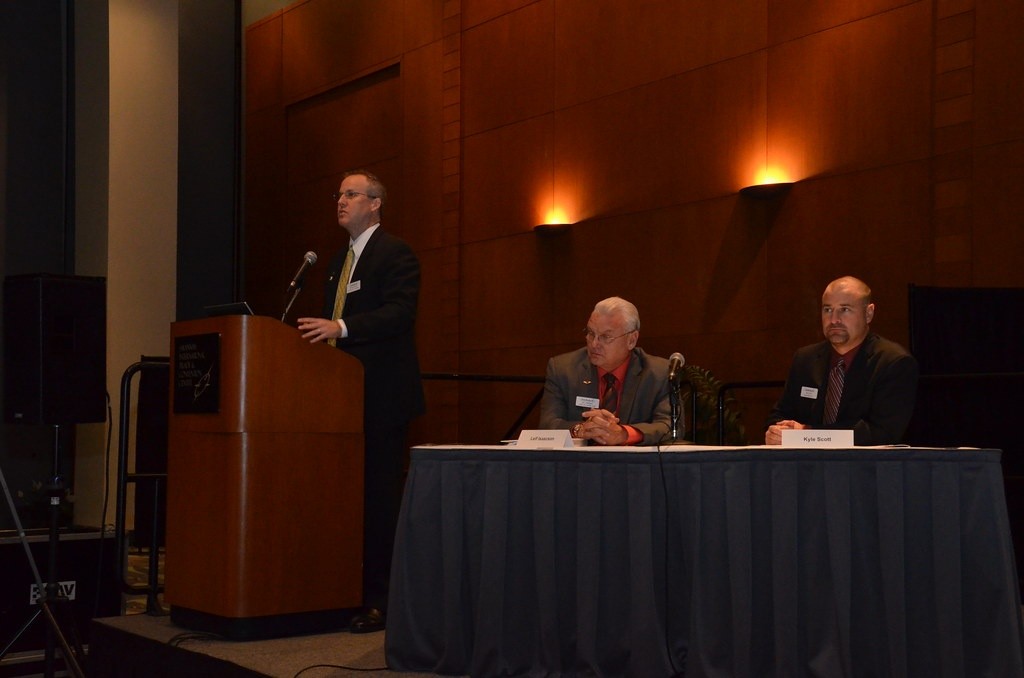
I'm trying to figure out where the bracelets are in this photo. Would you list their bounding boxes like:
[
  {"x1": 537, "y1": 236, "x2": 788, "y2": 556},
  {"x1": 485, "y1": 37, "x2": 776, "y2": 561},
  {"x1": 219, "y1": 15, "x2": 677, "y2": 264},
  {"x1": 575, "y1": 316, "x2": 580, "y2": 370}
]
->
[{"x1": 574, "y1": 424, "x2": 583, "y2": 437}]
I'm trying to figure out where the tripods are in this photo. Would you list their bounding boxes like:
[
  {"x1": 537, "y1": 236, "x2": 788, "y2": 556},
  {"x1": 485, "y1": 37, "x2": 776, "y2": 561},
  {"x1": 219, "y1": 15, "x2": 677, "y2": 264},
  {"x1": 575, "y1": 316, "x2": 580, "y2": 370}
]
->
[{"x1": 0, "y1": 425, "x2": 93, "y2": 678}]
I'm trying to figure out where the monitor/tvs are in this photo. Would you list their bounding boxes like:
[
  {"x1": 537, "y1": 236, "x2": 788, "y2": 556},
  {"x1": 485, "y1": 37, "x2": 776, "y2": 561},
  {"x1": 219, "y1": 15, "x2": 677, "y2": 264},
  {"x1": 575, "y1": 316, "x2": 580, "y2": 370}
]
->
[{"x1": 204, "y1": 302, "x2": 255, "y2": 315}]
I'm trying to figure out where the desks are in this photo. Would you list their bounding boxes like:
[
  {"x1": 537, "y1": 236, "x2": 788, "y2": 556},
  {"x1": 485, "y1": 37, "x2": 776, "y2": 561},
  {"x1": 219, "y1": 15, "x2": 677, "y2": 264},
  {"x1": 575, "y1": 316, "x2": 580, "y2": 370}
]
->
[{"x1": 384, "y1": 442, "x2": 1024, "y2": 678}]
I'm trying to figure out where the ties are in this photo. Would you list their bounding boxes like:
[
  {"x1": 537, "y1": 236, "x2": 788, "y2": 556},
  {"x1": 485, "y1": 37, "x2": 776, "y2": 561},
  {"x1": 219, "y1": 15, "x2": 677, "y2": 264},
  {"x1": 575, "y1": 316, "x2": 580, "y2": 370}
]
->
[
  {"x1": 822, "y1": 357, "x2": 845, "y2": 425},
  {"x1": 602, "y1": 373, "x2": 618, "y2": 412},
  {"x1": 327, "y1": 246, "x2": 354, "y2": 347}
]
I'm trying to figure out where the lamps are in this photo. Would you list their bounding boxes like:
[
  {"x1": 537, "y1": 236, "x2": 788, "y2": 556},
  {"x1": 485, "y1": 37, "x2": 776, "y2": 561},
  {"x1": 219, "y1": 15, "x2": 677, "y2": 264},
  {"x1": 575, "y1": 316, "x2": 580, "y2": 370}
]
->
[
  {"x1": 739, "y1": 183, "x2": 789, "y2": 200},
  {"x1": 532, "y1": 221, "x2": 572, "y2": 238}
]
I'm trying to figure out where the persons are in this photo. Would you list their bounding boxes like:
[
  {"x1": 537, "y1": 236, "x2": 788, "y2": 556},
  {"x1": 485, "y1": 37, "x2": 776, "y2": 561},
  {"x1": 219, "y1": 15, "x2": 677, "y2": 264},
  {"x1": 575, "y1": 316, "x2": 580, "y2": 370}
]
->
[
  {"x1": 765, "y1": 276, "x2": 920, "y2": 446},
  {"x1": 298, "y1": 172, "x2": 427, "y2": 631},
  {"x1": 540, "y1": 297, "x2": 686, "y2": 445}
]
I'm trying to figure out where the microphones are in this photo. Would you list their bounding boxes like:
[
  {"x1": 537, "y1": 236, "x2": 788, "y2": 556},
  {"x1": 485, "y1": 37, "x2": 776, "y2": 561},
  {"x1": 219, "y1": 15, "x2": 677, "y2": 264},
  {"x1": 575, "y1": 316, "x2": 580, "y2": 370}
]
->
[
  {"x1": 666, "y1": 353, "x2": 685, "y2": 382},
  {"x1": 285, "y1": 251, "x2": 318, "y2": 293}
]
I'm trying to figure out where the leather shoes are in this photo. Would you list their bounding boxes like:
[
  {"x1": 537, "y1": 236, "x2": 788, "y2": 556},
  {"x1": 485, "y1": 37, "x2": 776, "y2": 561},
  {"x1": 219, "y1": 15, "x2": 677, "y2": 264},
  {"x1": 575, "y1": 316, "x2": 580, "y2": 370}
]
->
[{"x1": 350, "y1": 608, "x2": 385, "y2": 632}]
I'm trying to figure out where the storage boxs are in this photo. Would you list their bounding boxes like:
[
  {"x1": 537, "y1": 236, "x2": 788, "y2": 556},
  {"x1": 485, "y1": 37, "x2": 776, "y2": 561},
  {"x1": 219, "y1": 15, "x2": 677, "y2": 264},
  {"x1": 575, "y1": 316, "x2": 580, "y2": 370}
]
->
[{"x1": 0, "y1": 525, "x2": 129, "y2": 652}]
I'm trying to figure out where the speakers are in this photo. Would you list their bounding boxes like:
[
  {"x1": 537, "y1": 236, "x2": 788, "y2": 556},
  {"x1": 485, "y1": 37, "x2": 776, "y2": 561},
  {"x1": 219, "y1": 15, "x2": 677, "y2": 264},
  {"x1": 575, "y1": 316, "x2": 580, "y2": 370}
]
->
[{"x1": 0, "y1": 275, "x2": 107, "y2": 425}]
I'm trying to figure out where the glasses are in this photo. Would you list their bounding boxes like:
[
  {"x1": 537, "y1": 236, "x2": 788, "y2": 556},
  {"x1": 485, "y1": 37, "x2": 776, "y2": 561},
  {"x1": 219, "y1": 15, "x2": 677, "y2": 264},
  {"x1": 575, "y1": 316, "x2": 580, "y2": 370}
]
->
[
  {"x1": 583, "y1": 327, "x2": 636, "y2": 344},
  {"x1": 333, "y1": 189, "x2": 379, "y2": 202}
]
